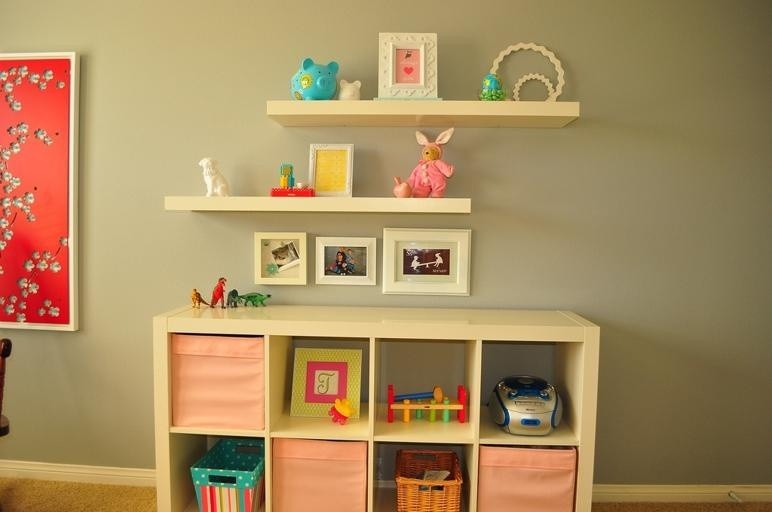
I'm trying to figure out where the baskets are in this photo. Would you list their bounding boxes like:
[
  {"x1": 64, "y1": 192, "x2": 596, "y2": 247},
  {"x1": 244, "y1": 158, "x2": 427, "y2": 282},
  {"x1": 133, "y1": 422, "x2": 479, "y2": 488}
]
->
[{"x1": 396, "y1": 449, "x2": 462, "y2": 511}]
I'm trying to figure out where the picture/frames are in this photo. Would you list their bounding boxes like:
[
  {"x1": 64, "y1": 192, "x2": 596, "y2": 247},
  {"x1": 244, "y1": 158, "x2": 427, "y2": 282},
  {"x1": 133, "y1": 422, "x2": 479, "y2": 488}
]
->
[
  {"x1": 0, "y1": 53, "x2": 78, "y2": 331},
  {"x1": 251, "y1": 228, "x2": 473, "y2": 297},
  {"x1": 377, "y1": 32, "x2": 439, "y2": 98}
]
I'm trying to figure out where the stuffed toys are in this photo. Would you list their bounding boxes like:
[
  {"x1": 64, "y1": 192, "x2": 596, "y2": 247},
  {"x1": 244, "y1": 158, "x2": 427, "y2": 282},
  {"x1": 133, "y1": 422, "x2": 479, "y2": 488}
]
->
[{"x1": 408, "y1": 127, "x2": 456, "y2": 198}]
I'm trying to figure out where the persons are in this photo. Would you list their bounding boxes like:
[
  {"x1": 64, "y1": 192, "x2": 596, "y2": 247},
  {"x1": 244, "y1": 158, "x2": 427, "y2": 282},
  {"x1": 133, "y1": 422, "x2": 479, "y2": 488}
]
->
[{"x1": 333, "y1": 248, "x2": 356, "y2": 275}]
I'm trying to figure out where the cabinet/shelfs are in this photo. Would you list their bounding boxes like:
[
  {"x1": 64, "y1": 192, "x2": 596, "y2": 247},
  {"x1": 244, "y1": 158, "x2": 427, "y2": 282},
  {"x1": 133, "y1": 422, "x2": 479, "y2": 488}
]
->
[
  {"x1": 152, "y1": 305, "x2": 599, "y2": 512},
  {"x1": 161, "y1": 98, "x2": 579, "y2": 214},
  {"x1": 307, "y1": 142, "x2": 353, "y2": 195}
]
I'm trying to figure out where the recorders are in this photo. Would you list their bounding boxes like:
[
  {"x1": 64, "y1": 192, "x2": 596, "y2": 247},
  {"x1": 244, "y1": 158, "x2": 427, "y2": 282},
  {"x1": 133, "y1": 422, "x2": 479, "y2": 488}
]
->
[{"x1": 488, "y1": 374, "x2": 563, "y2": 436}]
